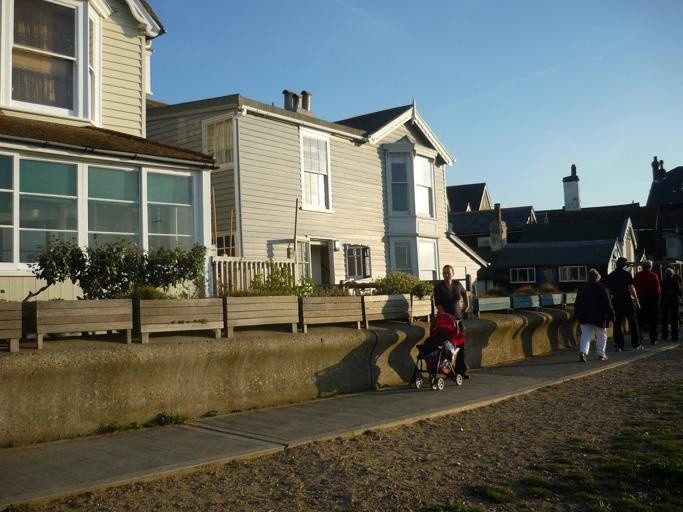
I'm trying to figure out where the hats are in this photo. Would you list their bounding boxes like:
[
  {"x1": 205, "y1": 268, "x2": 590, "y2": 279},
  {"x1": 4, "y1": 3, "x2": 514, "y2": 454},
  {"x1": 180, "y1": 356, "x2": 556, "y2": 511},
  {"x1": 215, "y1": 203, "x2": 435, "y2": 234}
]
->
[{"x1": 615, "y1": 257, "x2": 629, "y2": 267}]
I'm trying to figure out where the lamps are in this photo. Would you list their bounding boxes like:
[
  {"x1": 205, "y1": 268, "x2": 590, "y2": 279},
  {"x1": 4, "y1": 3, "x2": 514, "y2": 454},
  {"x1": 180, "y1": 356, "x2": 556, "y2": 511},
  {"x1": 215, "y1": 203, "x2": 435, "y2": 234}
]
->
[{"x1": 334, "y1": 240, "x2": 339, "y2": 251}]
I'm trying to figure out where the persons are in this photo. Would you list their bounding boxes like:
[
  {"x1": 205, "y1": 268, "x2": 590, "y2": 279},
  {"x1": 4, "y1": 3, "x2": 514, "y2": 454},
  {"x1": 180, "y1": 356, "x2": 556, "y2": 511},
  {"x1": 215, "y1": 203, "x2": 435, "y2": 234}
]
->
[
  {"x1": 574, "y1": 257, "x2": 682, "y2": 362},
  {"x1": 433, "y1": 264, "x2": 471, "y2": 379}
]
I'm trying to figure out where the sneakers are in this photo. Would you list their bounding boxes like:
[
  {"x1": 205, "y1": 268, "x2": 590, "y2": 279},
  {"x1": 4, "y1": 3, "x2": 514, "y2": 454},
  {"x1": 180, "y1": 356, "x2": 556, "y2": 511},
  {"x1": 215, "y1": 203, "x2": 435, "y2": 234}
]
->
[
  {"x1": 633, "y1": 334, "x2": 679, "y2": 351},
  {"x1": 598, "y1": 354, "x2": 608, "y2": 360},
  {"x1": 579, "y1": 353, "x2": 587, "y2": 362}
]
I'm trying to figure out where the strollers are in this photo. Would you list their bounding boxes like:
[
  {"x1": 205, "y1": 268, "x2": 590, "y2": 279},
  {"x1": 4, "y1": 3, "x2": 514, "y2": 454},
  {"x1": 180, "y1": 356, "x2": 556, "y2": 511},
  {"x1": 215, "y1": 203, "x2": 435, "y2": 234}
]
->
[{"x1": 408, "y1": 310, "x2": 465, "y2": 390}]
